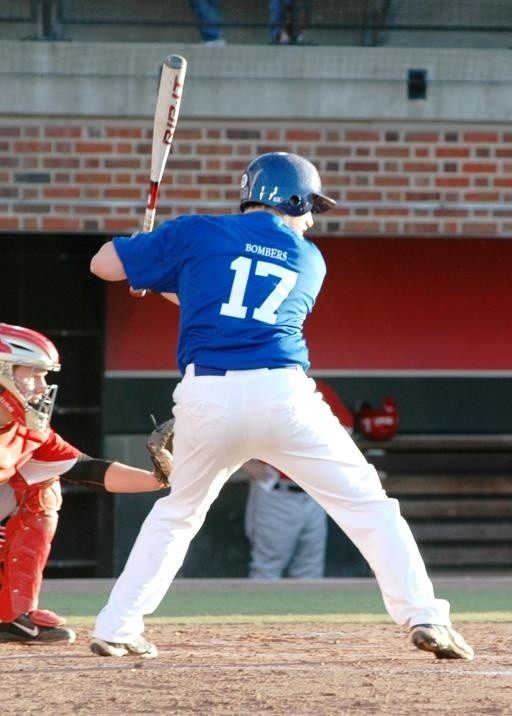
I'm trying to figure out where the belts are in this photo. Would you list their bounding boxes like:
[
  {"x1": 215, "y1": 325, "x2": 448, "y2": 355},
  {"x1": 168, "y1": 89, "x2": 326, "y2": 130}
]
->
[{"x1": 273, "y1": 483, "x2": 307, "y2": 492}]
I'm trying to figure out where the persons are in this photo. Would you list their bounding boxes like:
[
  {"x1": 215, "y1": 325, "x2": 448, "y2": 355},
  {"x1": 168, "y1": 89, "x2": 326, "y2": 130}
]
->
[
  {"x1": 238, "y1": 376, "x2": 356, "y2": 581},
  {"x1": 186, "y1": 0, "x2": 291, "y2": 45},
  {"x1": 88, "y1": 150, "x2": 478, "y2": 663},
  {"x1": 1, "y1": 320, "x2": 171, "y2": 648}
]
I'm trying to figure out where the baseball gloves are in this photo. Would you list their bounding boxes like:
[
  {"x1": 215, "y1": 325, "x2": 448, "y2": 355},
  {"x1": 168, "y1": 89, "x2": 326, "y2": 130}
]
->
[{"x1": 147, "y1": 414, "x2": 174, "y2": 489}]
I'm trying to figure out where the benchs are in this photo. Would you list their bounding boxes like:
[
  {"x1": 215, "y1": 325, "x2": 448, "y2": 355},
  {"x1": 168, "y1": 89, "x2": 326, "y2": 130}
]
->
[{"x1": 332, "y1": 428, "x2": 512, "y2": 575}]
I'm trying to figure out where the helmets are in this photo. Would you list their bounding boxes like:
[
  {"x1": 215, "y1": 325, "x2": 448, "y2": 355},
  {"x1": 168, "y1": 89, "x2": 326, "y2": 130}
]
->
[
  {"x1": 0, "y1": 323, "x2": 62, "y2": 433},
  {"x1": 355, "y1": 393, "x2": 397, "y2": 439},
  {"x1": 239, "y1": 150, "x2": 337, "y2": 218}
]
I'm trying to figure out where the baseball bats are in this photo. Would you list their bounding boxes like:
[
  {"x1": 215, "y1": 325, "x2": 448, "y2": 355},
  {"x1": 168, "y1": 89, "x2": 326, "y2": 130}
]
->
[{"x1": 128, "y1": 56, "x2": 187, "y2": 298}]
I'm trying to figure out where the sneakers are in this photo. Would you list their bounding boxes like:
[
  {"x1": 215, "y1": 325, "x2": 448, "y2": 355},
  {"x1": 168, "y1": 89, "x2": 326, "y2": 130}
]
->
[
  {"x1": 89, "y1": 636, "x2": 159, "y2": 659},
  {"x1": 409, "y1": 623, "x2": 475, "y2": 661},
  {"x1": 0, "y1": 612, "x2": 77, "y2": 646}
]
